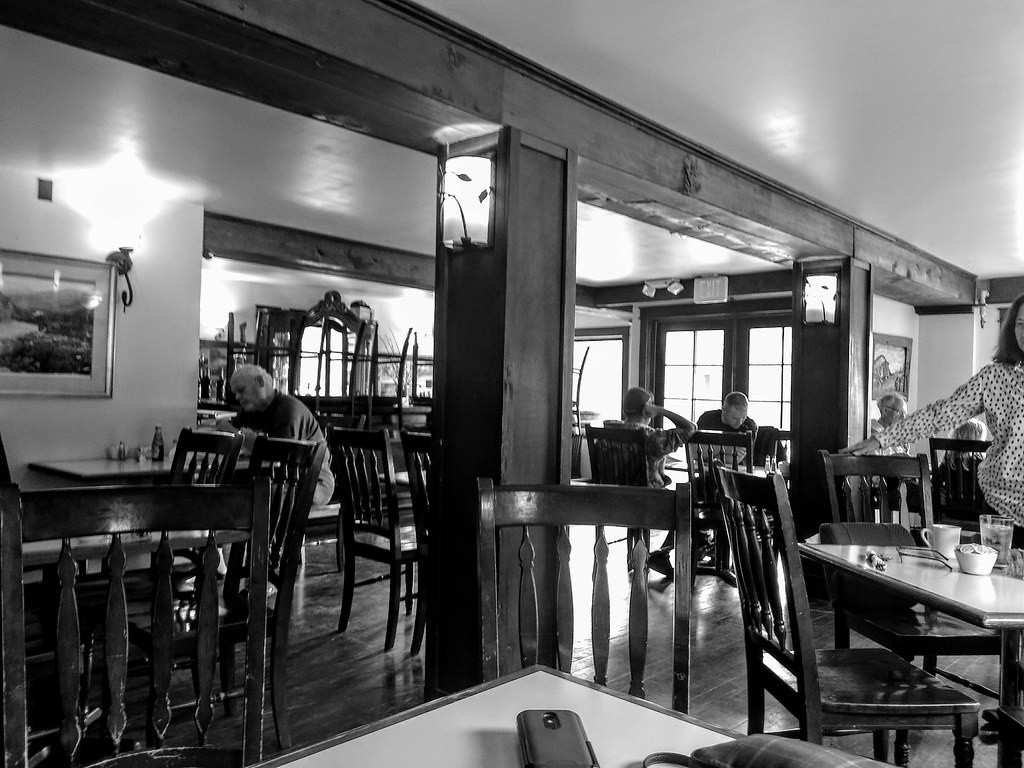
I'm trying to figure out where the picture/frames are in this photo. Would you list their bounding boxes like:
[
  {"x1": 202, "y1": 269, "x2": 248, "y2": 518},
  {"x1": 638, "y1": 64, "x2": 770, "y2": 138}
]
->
[{"x1": 0, "y1": 248, "x2": 118, "y2": 399}]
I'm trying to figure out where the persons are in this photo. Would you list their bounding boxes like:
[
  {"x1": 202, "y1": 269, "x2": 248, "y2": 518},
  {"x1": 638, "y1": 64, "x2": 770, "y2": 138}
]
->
[
  {"x1": 186, "y1": 365, "x2": 334, "y2": 599},
  {"x1": 840, "y1": 298, "x2": 1024, "y2": 726},
  {"x1": 598, "y1": 388, "x2": 697, "y2": 579},
  {"x1": 870, "y1": 393, "x2": 908, "y2": 488},
  {"x1": 928, "y1": 419, "x2": 989, "y2": 521},
  {"x1": 689, "y1": 392, "x2": 757, "y2": 564}
]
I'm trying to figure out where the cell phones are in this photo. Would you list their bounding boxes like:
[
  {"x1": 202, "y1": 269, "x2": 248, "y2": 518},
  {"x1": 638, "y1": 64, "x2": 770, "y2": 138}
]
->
[{"x1": 517, "y1": 710, "x2": 602, "y2": 768}]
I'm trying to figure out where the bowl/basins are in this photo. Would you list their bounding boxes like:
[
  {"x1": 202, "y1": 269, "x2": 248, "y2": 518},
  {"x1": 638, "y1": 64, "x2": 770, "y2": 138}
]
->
[{"x1": 954, "y1": 550, "x2": 998, "y2": 576}]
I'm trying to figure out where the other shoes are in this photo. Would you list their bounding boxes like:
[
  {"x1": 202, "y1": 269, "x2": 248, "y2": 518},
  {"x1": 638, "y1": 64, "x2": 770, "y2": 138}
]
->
[
  {"x1": 647, "y1": 550, "x2": 674, "y2": 576},
  {"x1": 706, "y1": 547, "x2": 715, "y2": 567}
]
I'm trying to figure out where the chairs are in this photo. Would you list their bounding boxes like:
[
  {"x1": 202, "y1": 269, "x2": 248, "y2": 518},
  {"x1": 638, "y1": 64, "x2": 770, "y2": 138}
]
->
[{"x1": 0, "y1": 312, "x2": 1024, "y2": 768}]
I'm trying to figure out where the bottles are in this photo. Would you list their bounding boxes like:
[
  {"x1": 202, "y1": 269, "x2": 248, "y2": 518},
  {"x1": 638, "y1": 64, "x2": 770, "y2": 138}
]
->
[
  {"x1": 169, "y1": 439, "x2": 178, "y2": 462},
  {"x1": 118, "y1": 441, "x2": 125, "y2": 460},
  {"x1": 764, "y1": 455, "x2": 777, "y2": 474},
  {"x1": 151, "y1": 425, "x2": 166, "y2": 463}
]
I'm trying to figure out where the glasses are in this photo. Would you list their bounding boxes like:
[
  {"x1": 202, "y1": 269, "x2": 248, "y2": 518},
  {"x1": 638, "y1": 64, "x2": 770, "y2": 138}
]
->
[
  {"x1": 896, "y1": 545, "x2": 952, "y2": 570},
  {"x1": 885, "y1": 405, "x2": 909, "y2": 417}
]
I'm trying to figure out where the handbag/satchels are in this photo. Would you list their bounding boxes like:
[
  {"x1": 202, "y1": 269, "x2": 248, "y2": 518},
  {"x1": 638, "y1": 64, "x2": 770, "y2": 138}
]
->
[{"x1": 641, "y1": 734, "x2": 899, "y2": 768}]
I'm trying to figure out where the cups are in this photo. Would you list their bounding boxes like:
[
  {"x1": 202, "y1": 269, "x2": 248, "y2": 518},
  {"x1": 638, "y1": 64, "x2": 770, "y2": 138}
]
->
[
  {"x1": 920, "y1": 524, "x2": 965, "y2": 559},
  {"x1": 979, "y1": 514, "x2": 1015, "y2": 568}
]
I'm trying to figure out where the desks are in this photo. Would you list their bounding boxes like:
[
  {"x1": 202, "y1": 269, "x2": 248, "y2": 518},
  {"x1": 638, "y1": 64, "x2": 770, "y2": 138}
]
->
[
  {"x1": 798, "y1": 543, "x2": 1024, "y2": 768},
  {"x1": 26, "y1": 456, "x2": 282, "y2": 482},
  {"x1": 241, "y1": 663, "x2": 750, "y2": 768}
]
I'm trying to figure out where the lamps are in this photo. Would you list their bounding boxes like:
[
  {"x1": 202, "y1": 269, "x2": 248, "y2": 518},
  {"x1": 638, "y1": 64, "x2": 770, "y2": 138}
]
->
[
  {"x1": 802, "y1": 273, "x2": 839, "y2": 326},
  {"x1": 107, "y1": 246, "x2": 133, "y2": 313},
  {"x1": 642, "y1": 278, "x2": 684, "y2": 297},
  {"x1": 441, "y1": 155, "x2": 494, "y2": 251}
]
